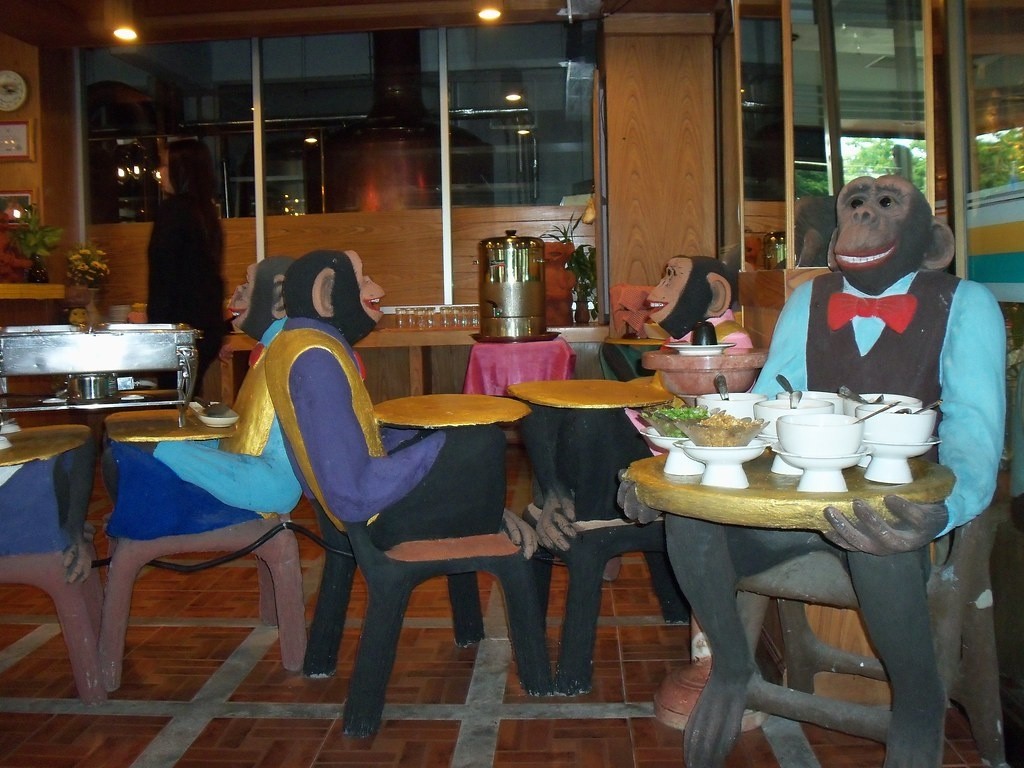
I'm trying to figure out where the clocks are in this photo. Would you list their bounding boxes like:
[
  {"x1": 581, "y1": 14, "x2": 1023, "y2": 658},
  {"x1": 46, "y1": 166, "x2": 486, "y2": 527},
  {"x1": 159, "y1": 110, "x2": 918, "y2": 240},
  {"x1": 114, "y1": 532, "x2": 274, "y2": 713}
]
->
[{"x1": 0, "y1": 70, "x2": 27, "y2": 111}]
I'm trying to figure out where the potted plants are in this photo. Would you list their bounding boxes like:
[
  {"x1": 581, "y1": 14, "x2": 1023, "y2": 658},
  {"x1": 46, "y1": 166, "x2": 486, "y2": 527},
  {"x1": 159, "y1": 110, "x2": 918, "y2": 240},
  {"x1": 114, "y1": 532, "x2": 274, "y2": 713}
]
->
[
  {"x1": 13, "y1": 203, "x2": 65, "y2": 283},
  {"x1": 538, "y1": 211, "x2": 595, "y2": 322}
]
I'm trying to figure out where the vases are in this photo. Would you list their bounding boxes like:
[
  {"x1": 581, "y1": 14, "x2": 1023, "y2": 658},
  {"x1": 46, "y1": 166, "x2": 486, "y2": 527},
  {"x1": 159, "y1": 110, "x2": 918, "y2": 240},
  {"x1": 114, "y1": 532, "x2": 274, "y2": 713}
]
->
[{"x1": 57, "y1": 288, "x2": 100, "y2": 308}]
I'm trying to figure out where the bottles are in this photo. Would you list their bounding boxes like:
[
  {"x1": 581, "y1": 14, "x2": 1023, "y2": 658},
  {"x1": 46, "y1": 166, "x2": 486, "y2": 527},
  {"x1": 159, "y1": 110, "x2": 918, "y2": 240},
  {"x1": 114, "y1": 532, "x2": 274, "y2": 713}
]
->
[{"x1": 128, "y1": 303, "x2": 148, "y2": 325}]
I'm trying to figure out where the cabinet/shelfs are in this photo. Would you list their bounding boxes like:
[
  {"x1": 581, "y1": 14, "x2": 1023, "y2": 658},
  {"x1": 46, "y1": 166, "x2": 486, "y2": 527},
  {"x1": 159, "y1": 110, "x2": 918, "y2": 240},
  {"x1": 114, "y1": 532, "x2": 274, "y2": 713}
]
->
[
  {"x1": 420, "y1": 23, "x2": 568, "y2": 72},
  {"x1": 211, "y1": 23, "x2": 375, "y2": 84}
]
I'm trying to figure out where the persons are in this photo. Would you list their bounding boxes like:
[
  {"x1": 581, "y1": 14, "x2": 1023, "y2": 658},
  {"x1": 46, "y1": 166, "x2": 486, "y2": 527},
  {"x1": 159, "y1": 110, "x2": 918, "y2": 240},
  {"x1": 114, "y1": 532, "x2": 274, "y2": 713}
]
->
[{"x1": 146, "y1": 135, "x2": 226, "y2": 401}]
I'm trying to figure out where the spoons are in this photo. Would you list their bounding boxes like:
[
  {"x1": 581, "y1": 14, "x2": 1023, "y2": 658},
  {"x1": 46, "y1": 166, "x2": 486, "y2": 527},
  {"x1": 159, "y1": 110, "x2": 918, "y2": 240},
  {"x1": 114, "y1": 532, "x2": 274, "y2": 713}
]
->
[
  {"x1": 894, "y1": 398, "x2": 944, "y2": 415},
  {"x1": 653, "y1": 411, "x2": 673, "y2": 423},
  {"x1": 839, "y1": 385, "x2": 884, "y2": 404},
  {"x1": 713, "y1": 374, "x2": 730, "y2": 400},
  {"x1": 194, "y1": 396, "x2": 231, "y2": 418},
  {"x1": 789, "y1": 388, "x2": 803, "y2": 409},
  {"x1": 841, "y1": 401, "x2": 902, "y2": 425},
  {"x1": 776, "y1": 373, "x2": 797, "y2": 394}
]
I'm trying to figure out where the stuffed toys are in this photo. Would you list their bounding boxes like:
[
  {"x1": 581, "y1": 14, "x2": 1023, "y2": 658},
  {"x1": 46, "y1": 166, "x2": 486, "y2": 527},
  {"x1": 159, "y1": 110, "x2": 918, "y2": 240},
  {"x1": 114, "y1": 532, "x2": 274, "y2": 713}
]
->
[
  {"x1": 616, "y1": 173, "x2": 1010, "y2": 768},
  {"x1": 264, "y1": 249, "x2": 547, "y2": 559},
  {"x1": 100, "y1": 256, "x2": 303, "y2": 543},
  {"x1": 516, "y1": 252, "x2": 740, "y2": 554},
  {"x1": 0, "y1": 430, "x2": 102, "y2": 585}
]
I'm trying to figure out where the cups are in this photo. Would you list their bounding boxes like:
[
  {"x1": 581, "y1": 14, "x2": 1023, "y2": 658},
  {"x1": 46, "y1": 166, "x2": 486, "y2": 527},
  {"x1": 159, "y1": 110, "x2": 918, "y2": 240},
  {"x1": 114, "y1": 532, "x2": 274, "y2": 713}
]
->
[
  {"x1": 691, "y1": 321, "x2": 718, "y2": 345},
  {"x1": 395, "y1": 307, "x2": 480, "y2": 328}
]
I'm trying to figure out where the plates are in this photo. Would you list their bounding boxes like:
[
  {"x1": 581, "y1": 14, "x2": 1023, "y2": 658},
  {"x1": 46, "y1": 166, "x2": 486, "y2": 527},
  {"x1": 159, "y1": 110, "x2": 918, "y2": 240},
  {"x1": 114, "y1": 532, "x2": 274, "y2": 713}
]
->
[
  {"x1": 672, "y1": 414, "x2": 770, "y2": 447},
  {"x1": 637, "y1": 404, "x2": 721, "y2": 437},
  {"x1": 665, "y1": 343, "x2": 737, "y2": 355},
  {"x1": 189, "y1": 400, "x2": 239, "y2": 427},
  {"x1": 109, "y1": 305, "x2": 130, "y2": 321}
]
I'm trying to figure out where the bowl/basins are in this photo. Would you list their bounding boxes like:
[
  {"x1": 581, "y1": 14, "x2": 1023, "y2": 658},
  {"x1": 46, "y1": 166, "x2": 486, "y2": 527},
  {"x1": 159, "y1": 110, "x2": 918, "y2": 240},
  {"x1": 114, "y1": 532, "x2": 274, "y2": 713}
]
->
[
  {"x1": 774, "y1": 414, "x2": 865, "y2": 457},
  {"x1": 696, "y1": 392, "x2": 768, "y2": 423},
  {"x1": 776, "y1": 391, "x2": 844, "y2": 414},
  {"x1": 754, "y1": 400, "x2": 835, "y2": 435},
  {"x1": 0, "y1": 436, "x2": 13, "y2": 450},
  {"x1": 844, "y1": 393, "x2": 923, "y2": 417},
  {"x1": 855, "y1": 404, "x2": 937, "y2": 442},
  {"x1": 0, "y1": 425, "x2": 21, "y2": 435}
]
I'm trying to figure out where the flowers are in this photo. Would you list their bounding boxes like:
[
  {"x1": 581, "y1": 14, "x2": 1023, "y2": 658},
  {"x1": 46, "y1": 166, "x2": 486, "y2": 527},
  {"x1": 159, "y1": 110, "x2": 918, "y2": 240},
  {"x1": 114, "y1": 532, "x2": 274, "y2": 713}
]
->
[{"x1": 66, "y1": 243, "x2": 110, "y2": 286}]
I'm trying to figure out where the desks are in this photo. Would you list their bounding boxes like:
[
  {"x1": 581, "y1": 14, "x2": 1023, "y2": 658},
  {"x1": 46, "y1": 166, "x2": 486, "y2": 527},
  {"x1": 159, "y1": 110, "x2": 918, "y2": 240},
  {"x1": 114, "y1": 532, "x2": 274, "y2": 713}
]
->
[
  {"x1": 0, "y1": 176, "x2": 1024, "y2": 768},
  {"x1": 466, "y1": 338, "x2": 576, "y2": 444},
  {"x1": 221, "y1": 320, "x2": 615, "y2": 407}
]
